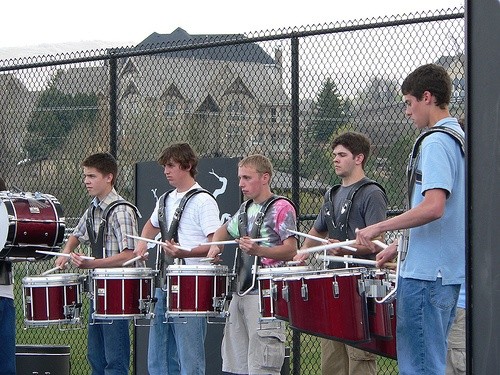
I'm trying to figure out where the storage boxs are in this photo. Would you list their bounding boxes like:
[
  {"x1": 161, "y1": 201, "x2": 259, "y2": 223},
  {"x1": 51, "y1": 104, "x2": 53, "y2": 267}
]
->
[{"x1": 15, "y1": 344, "x2": 70, "y2": 375}]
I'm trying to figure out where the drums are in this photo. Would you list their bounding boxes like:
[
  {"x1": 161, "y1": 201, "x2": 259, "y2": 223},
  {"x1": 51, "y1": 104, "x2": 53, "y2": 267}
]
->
[
  {"x1": 0, "y1": 190, "x2": 66, "y2": 263},
  {"x1": 164, "y1": 264, "x2": 231, "y2": 319},
  {"x1": 21, "y1": 272, "x2": 85, "y2": 326},
  {"x1": 268, "y1": 268, "x2": 307, "y2": 322},
  {"x1": 350, "y1": 276, "x2": 398, "y2": 361},
  {"x1": 255, "y1": 264, "x2": 310, "y2": 321},
  {"x1": 91, "y1": 266, "x2": 157, "y2": 320},
  {"x1": 282, "y1": 265, "x2": 371, "y2": 344},
  {"x1": 365, "y1": 270, "x2": 393, "y2": 339}
]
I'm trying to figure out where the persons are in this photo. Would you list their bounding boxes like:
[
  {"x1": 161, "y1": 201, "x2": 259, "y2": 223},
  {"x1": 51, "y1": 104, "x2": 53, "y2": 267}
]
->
[
  {"x1": 207, "y1": 157, "x2": 298, "y2": 375},
  {"x1": 356, "y1": 63, "x2": 466, "y2": 375},
  {"x1": 55, "y1": 152, "x2": 139, "y2": 375},
  {"x1": 132, "y1": 143, "x2": 224, "y2": 375},
  {"x1": 293, "y1": 131, "x2": 389, "y2": 375},
  {"x1": 0, "y1": 177, "x2": 17, "y2": 375}
]
